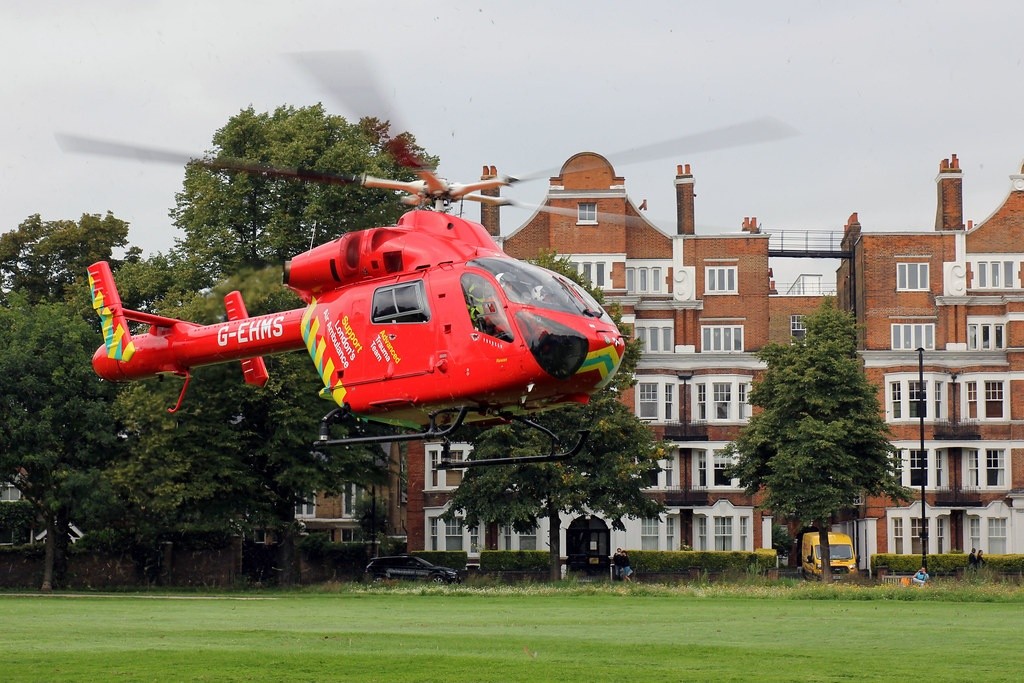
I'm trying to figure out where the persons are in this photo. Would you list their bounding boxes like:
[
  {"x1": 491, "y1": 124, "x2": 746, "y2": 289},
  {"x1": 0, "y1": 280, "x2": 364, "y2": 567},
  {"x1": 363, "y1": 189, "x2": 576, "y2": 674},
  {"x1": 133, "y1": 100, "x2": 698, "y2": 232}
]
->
[
  {"x1": 484, "y1": 272, "x2": 557, "y2": 353},
  {"x1": 977, "y1": 550, "x2": 986, "y2": 570},
  {"x1": 613, "y1": 548, "x2": 623, "y2": 583},
  {"x1": 621, "y1": 550, "x2": 633, "y2": 584},
  {"x1": 912, "y1": 567, "x2": 930, "y2": 587},
  {"x1": 969, "y1": 548, "x2": 977, "y2": 584}
]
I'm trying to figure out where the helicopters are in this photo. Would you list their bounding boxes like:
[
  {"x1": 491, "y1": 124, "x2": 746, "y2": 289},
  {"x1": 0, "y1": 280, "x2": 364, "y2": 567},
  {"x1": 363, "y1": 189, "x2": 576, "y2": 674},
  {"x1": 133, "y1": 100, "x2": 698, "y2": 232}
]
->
[{"x1": 51, "y1": 45, "x2": 802, "y2": 468}]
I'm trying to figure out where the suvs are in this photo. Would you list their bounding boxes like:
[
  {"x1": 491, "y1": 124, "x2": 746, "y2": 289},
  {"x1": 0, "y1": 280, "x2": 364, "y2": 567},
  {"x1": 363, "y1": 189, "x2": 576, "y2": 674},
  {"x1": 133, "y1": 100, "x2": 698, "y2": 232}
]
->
[{"x1": 365, "y1": 555, "x2": 460, "y2": 587}]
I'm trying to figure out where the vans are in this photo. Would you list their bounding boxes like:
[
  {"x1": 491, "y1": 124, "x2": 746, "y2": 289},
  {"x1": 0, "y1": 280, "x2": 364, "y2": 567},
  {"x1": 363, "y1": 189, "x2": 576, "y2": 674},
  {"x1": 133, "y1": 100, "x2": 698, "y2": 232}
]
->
[{"x1": 803, "y1": 529, "x2": 861, "y2": 585}]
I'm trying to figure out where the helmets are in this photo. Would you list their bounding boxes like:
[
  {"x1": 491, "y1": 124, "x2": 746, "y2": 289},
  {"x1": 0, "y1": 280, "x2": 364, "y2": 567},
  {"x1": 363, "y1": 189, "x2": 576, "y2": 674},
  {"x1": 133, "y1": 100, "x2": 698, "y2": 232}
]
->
[
  {"x1": 495, "y1": 273, "x2": 517, "y2": 290},
  {"x1": 531, "y1": 285, "x2": 554, "y2": 302}
]
